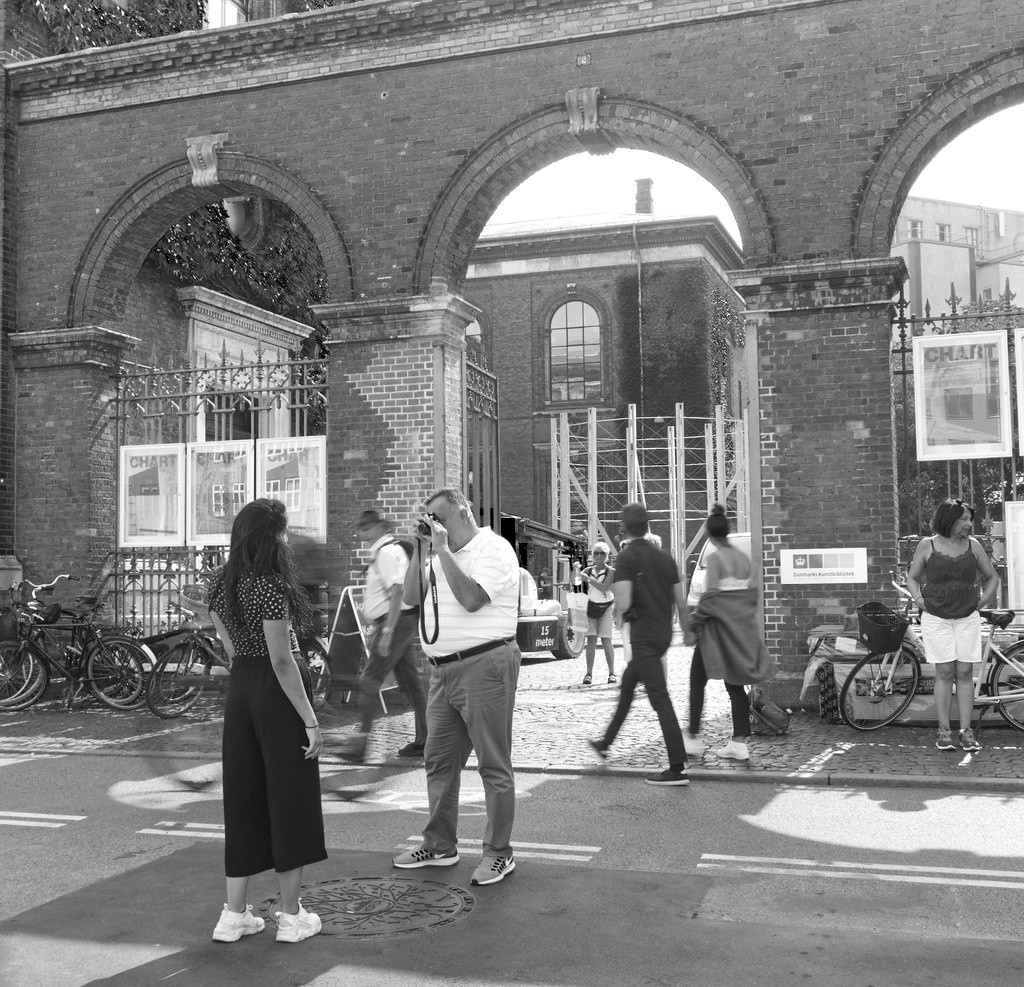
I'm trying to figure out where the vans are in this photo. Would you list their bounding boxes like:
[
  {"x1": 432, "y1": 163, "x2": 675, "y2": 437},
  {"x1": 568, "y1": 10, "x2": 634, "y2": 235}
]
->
[{"x1": 687, "y1": 532, "x2": 751, "y2": 613}]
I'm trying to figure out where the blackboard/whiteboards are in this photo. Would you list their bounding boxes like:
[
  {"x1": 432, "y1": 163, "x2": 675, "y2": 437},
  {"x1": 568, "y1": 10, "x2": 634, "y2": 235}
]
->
[{"x1": 314, "y1": 585, "x2": 402, "y2": 692}]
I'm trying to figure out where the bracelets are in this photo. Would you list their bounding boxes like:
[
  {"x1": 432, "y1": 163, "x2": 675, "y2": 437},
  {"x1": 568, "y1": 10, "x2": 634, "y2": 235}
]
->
[
  {"x1": 304, "y1": 723, "x2": 320, "y2": 729},
  {"x1": 915, "y1": 597, "x2": 921, "y2": 601}
]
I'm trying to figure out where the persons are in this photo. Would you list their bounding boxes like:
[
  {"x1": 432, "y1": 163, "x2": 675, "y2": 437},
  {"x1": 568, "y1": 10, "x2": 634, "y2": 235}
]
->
[
  {"x1": 331, "y1": 509, "x2": 431, "y2": 764},
  {"x1": 391, "y1": 488, "x2": 523, "y2": 886},
  {"x1": 538, "y1": 568, "x2": 553, "y2": 600},
  {"x1": 208, "y1": 498, "x2": 329, "y2": 944},
  {"x1": 570, "y1": 505, "x2": 779, "y2": 786},
  {"x1": 907, "y1": 498, "x2": 999, "y2": 751}
]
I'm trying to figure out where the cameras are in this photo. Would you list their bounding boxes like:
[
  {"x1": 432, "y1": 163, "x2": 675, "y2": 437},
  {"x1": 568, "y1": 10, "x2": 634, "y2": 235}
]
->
[{"x1": 418, "y1": 512, "x2": 440, "y2": 536}]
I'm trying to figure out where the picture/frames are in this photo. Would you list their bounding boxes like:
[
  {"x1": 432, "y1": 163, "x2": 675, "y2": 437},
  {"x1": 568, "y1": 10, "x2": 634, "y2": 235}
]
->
[
  {"x1": 120, "y1": 436, "x2": 326, "y2": 550},
  {"x1": 912, "y1": 329, "x2": 1013, "y2": 461}
]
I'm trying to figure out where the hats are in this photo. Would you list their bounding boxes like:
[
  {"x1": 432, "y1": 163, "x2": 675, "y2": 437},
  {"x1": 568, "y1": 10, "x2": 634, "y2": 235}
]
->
[{"x1": 350, "y1": 509, "x2": 389, "y2": 531}]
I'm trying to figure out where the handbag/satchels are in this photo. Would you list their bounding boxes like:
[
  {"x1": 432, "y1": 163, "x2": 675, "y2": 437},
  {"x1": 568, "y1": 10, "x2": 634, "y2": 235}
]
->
[
  {"x1": 586, "y1": 598, "x2": 615, "y2": 618},
  {"x1": 746, "y1": 685, "x2": 790, "y2": 736}
]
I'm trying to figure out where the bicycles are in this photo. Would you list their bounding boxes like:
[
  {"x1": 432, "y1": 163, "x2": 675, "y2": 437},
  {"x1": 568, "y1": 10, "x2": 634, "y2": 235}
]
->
[
  {"x1": 0, "y1": 574, "x2": 159, "y2": 711},
  {"x1": 840, "y1": 570, "x2": 1024, "y2": 734},
  {"x1": 144, "y1": 583, "x2": 334, "y2": 719}
]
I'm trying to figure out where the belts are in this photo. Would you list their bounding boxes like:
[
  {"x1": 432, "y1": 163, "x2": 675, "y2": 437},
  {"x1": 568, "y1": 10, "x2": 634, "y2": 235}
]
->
[
  {"x1": 374, "y1": 607, "x2": 419, "y2": 625},
  {"x1": 428, "y1": 635, "x2": 518, "y2": 666}
]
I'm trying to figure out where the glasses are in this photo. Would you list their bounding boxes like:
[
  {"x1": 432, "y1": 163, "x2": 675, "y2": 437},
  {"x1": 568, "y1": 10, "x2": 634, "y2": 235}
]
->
[
  {"x1": 594, "y1": 551, "x2": 606, "y2": 556},
  {"x1": 950, "y1": 498, "x2": 962, "y2": 510}
]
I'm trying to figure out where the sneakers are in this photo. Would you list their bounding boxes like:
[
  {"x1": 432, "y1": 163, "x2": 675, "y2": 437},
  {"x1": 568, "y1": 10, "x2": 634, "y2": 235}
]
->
[
  {"x1": 682, "y1": 733, "x2": 705, "y2": 755},
  {"x1": 392, "y1": 846, "x2": 459, "y2": 868},
  {"x1": 275, "y1": 897, "x2": 322, "y2": 942},
  {"x1": 958, "y1": 727, "x2": 982, "y2": 751},
  {"x1": 589, "y1": 738, "x2": 609, "y2": 761},
  {"x1": 470, "y1": 857, "x2": 515, "y2": 885},
  {"x1": 935, "y1": 724, "x2": 955, "y2": 751},
  {"x1": 716, "y1": 741, "x2": 750, "y2": 760},
  {"x1": 212, "y1": 903, "x2": 266, "y2": 942},
  {"x1": 645, "y1": 770, "x2": 690, "y2": 785}
]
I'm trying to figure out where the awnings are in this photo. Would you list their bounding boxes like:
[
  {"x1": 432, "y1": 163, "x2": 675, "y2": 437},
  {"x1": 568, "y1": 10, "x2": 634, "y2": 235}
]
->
[{"x1": 522, "y1": 519, "x2": 584, "y2": 551}]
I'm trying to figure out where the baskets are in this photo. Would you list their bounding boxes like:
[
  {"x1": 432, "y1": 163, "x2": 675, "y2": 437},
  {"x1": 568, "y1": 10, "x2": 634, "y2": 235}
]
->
[{"x1": 856, "y1": 601, "x2": 909, "y2": 652}]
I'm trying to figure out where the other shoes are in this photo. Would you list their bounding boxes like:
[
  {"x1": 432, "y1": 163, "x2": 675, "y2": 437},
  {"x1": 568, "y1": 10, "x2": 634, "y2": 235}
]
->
[
  {"x1": 583, "y1": 675, "x2": 592, "y2": 684},
  {"x1": 399, "y1": 742, "x2": 427, "y2": 756},
  {"x1": 608, "y1": 672, "x2": 616, "y2": 683},
  {"x1": 334, "y1": 750, "x2": 365, "y2": 764}
]
